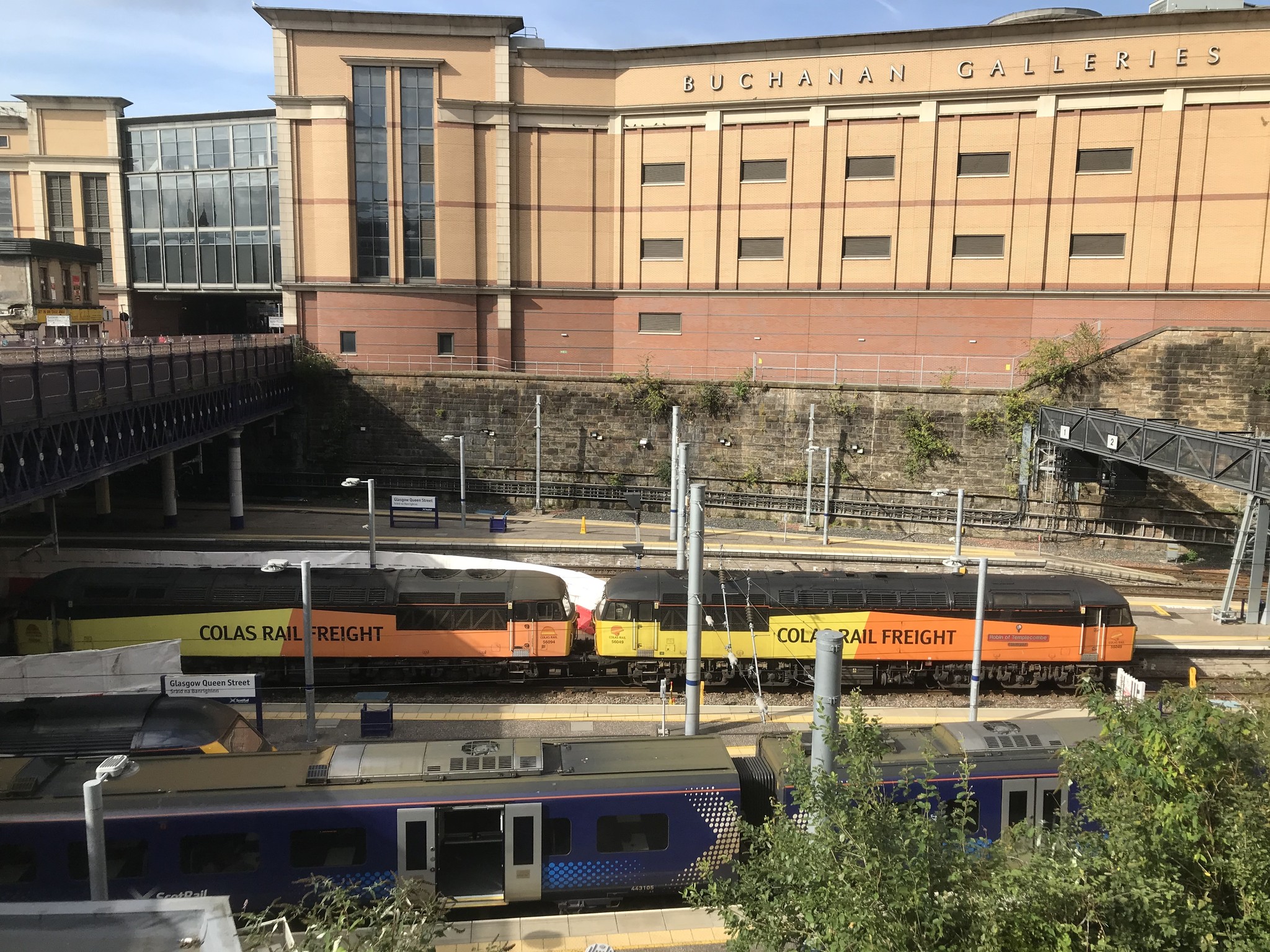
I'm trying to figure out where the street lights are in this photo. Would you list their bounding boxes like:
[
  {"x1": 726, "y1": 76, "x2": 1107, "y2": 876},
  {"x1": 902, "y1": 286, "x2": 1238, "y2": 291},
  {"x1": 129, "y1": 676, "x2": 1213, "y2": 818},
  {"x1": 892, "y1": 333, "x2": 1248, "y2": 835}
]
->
[
  {"x1": 341, "y1": 477, "x2": 377, "y2": 568},
  {"x1": 260, "y1": 559, "x2": 317, "y2": 742},
  {"x1": 441, "y1": 435, "x2": 466, "y2": 528},
  {"x1": 622, "y1": 491, "x2": 645, "y2": 571},
  {"x1": 83, "y1": 755, "x2": 130, "y2": 901}
]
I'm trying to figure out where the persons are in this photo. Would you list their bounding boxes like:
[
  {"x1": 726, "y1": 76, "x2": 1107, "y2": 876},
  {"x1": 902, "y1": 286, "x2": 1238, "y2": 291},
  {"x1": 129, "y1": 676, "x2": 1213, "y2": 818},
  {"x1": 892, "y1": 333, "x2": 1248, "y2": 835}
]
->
[{"x1": 144, "y1": 336, "x2": 150, "y2": 344}]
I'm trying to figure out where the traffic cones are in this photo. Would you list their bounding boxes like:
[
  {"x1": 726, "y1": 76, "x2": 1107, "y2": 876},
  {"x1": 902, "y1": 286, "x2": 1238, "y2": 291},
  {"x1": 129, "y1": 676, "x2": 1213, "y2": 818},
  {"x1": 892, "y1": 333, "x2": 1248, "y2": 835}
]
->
[{"x1": 580, "y1": 516, "x2": 587, "y2": 534}]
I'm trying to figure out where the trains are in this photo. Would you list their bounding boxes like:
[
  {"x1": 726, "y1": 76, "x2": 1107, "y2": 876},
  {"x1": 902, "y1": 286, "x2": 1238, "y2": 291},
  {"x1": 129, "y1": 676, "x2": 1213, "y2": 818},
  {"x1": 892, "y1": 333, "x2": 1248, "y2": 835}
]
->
[
  {"x1": 1, "y1": 549, "x2": 1140, "y2": 696},
  {"x1": 1, "y1": 721, "x2": 1116, "y2": 930},
  {"x1": 0, "y1": 697, "x2": 279, "y2": 757}
]
